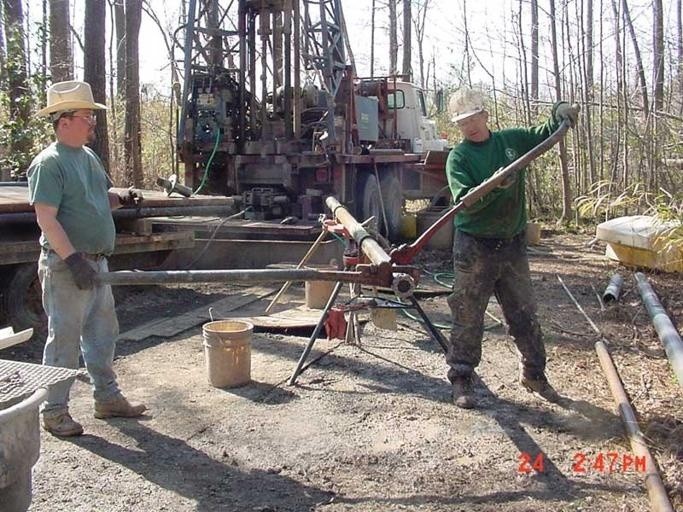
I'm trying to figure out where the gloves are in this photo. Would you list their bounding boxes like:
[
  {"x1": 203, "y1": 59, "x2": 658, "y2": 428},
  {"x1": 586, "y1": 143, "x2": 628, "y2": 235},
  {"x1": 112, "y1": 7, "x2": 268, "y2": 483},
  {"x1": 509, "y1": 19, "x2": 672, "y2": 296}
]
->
[
  {"x1": 489, "y1": 167, "x2": 516, "y2": 189},
  {"x1": 555, "y1": 103, "x2": 578, "y2": 129},
  {"x1": 119, "y1": 186, "x2": 142, "y2": 211},
  {"x1": 64, "y1": 252, "x2": 94, "y2": 290}
]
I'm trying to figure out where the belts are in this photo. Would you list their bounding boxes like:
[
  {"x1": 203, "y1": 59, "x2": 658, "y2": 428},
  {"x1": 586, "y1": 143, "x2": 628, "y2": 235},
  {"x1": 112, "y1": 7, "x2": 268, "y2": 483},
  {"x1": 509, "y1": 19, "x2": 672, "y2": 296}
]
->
[{"x1": 40, "y1": 248, "x2": 107, "y2": 261}]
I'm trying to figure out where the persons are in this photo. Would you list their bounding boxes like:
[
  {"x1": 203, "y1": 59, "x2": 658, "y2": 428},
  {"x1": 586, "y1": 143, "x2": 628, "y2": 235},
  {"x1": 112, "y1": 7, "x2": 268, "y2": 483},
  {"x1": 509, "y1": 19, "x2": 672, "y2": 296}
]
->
[
  {"x1": 26, "y1": 78, "x2": 145, "y2": 438},
  {"x1": 445, "y1": 87, "x2": 580, "y2": 410}
]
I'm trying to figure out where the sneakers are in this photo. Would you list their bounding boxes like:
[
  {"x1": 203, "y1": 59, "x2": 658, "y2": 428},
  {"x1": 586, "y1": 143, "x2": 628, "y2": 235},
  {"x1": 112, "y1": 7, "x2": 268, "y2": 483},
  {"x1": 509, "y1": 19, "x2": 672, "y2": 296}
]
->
[
  {"x1": 93, "y1": 398, "x2": 144, "y2": 416},
  {"x1": 41, "y1": 411, "x2": 84, "y2": 437},
  {"x1": 451, "y1": 378, "x2": 473, "y2": 408},
  {"x1": 518, "y1": 374, "x2": 558, "y2": 404}
]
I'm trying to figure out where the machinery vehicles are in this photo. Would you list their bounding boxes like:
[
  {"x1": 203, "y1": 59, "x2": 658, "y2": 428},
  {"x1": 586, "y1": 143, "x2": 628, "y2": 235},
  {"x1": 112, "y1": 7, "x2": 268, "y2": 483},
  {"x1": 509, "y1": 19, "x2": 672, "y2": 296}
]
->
[
  {"x1": 171, "y1": 1, "x2": 454, "y2": 268},
  {"x1": 0, "y1": 185, "x2": 236, "y2": 353}
]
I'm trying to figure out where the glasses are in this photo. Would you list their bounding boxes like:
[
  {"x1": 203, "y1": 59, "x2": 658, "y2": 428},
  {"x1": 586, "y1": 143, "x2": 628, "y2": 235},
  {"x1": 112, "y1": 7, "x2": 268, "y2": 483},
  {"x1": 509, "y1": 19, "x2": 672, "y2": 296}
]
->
[{"x1": 63, "y1": 114, "x2": 97, "y2": 123}]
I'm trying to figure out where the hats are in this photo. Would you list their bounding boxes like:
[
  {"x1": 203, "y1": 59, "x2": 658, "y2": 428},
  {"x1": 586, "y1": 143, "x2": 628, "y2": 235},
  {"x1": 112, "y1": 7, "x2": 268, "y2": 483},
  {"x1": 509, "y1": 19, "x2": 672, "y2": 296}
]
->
[
  {"x1": 449, "y1": 86, "x2": 486, "y2": 123},
  {"x1": 33, "y1": 81, "x2": 109, "y2": 118}
]
